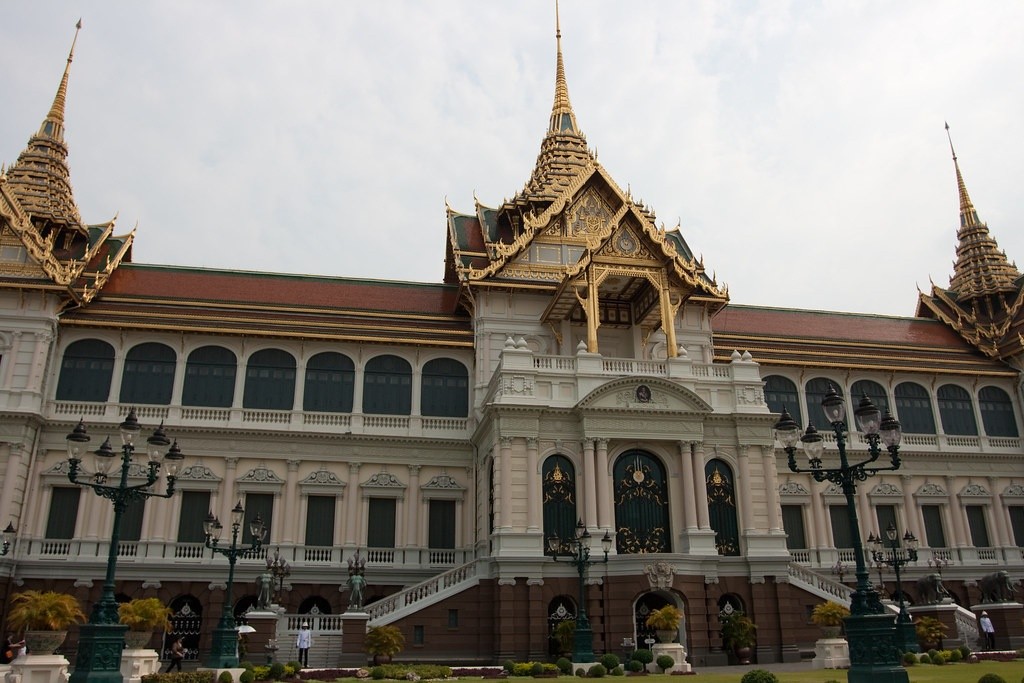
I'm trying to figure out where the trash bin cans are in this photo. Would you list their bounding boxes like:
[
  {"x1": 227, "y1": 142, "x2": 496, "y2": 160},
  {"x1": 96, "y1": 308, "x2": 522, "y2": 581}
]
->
[
  {"x1": 620, "y1": 643, "x2": 636, "y2": 664},
  {"x1": 9, "y1": 644, "x2": 22, "y2": 662}
]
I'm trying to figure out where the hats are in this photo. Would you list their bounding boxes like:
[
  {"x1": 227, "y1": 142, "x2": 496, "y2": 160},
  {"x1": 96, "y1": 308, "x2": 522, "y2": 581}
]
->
[
  {"x1": 982, "y1": 611, "x2": 987, "y2": 615},
  {"x1": 302, "y1": 622, "x2": 308, "y2": 626},
  {"x1": 19, "y1": 640, "x2": 25, "y2": 643}
]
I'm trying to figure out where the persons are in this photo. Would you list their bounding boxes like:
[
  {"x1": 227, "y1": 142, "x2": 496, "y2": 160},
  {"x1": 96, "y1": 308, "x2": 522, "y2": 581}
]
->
[
  {"x1": 165, "y1": 638, "x2": 185, "y2": 673},
  {"x1": 0, "y1": 634, "x2": 27, "y2": 664},
  {"x1": 980, "y1": 610, "x2": 995, "y2": 651},
  {"x1": 238, "y1": 640, "x2": 246, "y2": 662},
  {"x1": 296, "y1": 621, "x2": 311, "y2": 667}
]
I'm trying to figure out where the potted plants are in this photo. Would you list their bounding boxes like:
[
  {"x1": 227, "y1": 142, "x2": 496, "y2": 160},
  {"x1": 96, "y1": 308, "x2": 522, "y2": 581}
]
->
[
  {"x1": 810, "y1": 602, "x2": 847, "y2": 638},
  {"x1": 555, "y1": 621, "x2": 575, "y2": 662},
  {"x1": 647, "y1": 604, "x2": 683, "y2": 644},
  {"x1": 119, "y1": 597, "x2": 174, "y2": 649},
  {"x1": 6, "y1": 590, "x2": 89, "y2": 654},
  {"x1": 725, "y1": 610, "x2": 759, "y2": 665},
  {"x1": 916, "y1": 615, "x2": 948, "y2": 651},
  {"x1": 365, "y1": 625, "x2": 403, "y2": 665}
]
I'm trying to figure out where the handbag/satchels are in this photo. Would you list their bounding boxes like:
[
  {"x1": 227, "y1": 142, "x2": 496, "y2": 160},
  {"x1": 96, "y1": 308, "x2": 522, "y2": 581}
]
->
[{"x1": 6, "y1": 650, "x2": 13, "y2": 658}]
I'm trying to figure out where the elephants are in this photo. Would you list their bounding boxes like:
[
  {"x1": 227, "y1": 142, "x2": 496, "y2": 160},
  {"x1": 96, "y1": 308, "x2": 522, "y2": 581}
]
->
[
  {"x1": 345, "y1": 574, "x2": 367, "y2": 613},
  {"x1": 916, "y1": 573, "x2": 950, "y2": 605},
  {"x1": 980, "y1": 569, "x2": 1019, "y2": 604},
  {"x1": 255, "y1": 572, "x2": 276, "y2": 612}
]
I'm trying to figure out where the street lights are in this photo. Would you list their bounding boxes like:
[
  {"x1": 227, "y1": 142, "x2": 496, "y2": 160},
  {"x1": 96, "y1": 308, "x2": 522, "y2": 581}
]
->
[
  {"x1": 865, "y1": 520, "x2": 923, "y2": 655},
  {"x1": 346, "y1": 550, "x2": 367, "y2": 612},
  {"x1": 201, "y1": 497, "x2": 267, "y2": 667},
  {"x1": 267, "y1": 544, "x2": 290, "y2": 605},
  {"x1": 65, "y1": 404, "x2": 185, "y2": 683},
  {"x1": 770, "y1": 382, "x2": 910, "y2": 683},
  {"x1": 548, "y1": 517, "x2": 613, "y2": 663},
  {"x1": 927, "y1": 552, "x2": 948, "y2": 580},
  {"x1": 832, "y1": 558, "x2": 850, "y2": 585}
]
